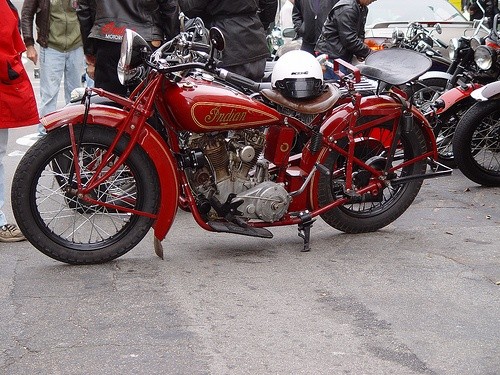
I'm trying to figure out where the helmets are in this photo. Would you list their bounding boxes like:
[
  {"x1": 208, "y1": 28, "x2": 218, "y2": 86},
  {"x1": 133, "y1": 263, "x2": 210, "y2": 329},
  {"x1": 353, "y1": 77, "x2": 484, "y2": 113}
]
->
[{"x1": 271, "y1": 50, "x2": 329, "y2": 99}]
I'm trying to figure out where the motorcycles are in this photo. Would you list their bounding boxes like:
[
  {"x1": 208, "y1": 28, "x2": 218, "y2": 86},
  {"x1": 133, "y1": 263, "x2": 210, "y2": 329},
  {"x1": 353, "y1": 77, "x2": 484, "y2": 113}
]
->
[
  {"x1": 264, "y1": 0, "x2": 500, "y2": 188},
  {"x1": 10, "y1": 12, "x2": 453, "y2": 266}
]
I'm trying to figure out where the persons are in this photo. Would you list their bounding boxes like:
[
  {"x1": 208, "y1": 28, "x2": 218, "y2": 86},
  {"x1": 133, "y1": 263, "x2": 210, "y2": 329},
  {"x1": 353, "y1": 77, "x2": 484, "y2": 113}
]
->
[
  {"x1": 176, "y1": 0, "x2": 278, "y2": 82},
  {"x1": 313, "y1": 0, "x2": 376, "y2": 80},
  {"x1": 291, "y1": 0, "x2": 340, "y2": 54},
  {"x1": 21, "y1": 0, "x2": 85, "y2": 138},
  {"x1": 0, "y1": 0, "x2": 40, "y2": 243},
  {"x1": 76, "y1": 0, "x2": 180, "y2": 145}
]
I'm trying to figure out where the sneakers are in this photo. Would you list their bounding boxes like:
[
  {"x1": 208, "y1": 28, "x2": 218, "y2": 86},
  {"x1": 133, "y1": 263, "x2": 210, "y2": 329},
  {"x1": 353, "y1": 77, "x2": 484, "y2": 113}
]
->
[{"x1": 0, "y1": 224, "x2": 26, "y2": 241}]
